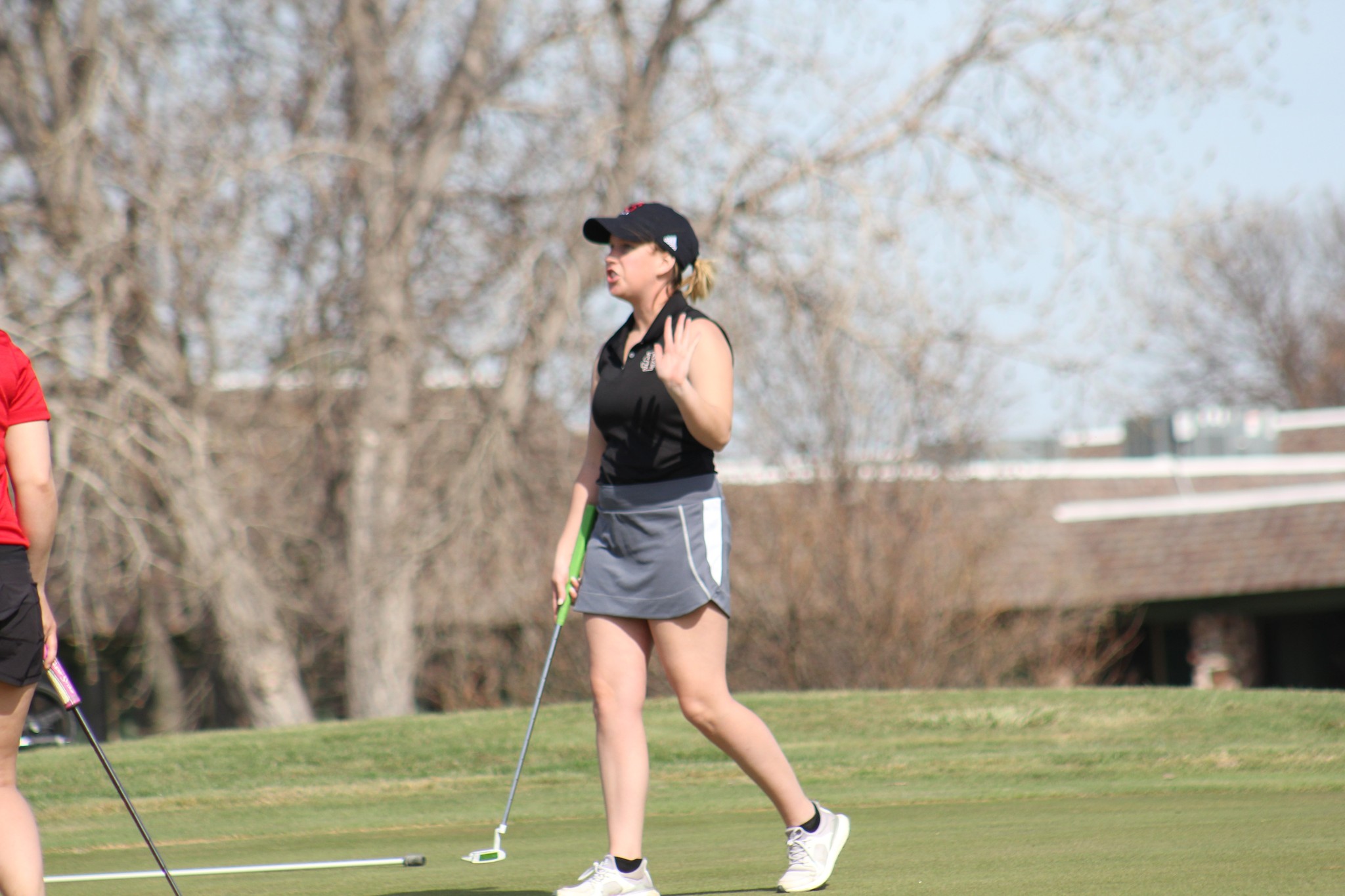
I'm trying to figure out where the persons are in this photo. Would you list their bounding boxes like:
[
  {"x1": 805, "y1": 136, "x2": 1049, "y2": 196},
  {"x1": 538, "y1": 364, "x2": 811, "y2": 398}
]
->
[
  {"x1": 554, "y1": 201, "x2": 851, "y2": 896},
  {"x1": 0, "y1": 329, "x2": 56, "y2": 896}
]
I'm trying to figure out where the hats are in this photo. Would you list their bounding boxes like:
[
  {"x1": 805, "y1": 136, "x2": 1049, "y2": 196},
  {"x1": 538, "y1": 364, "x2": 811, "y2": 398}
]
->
[{"x1": 584, "y1": 202, "x2": 699, "y2": 269}]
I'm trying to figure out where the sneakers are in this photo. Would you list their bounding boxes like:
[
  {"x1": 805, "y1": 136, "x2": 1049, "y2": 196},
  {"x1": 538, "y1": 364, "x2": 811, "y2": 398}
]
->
[
  {"x1": 555, "y1": 855, "x2": 660, "y2": 896},
  {"x1": 776, "y1": 801, "x2": 850, "y2": 892}
]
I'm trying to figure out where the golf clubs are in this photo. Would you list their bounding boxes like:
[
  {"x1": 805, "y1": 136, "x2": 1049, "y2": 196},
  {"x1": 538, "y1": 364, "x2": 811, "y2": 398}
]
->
[
  {"x1": 40, "y1": 853, "x2": 426, "y2": 883},
  {"x1": 459, "y1": 504, "x2": 601, "y2": 865},
  {"x1": 41, "y1": 642, "x2": 182, "y2": 896}
]
